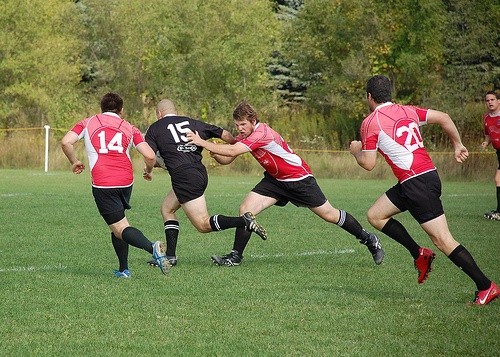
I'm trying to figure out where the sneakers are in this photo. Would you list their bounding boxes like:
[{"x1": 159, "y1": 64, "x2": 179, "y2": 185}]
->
[
  {"x1": 414, "y1": 248, "x2": 436, "y2": 283},
  {"x1": 241, "y1": 212, "x2": 267, "y2": 240},
  {"x1": 359, "y1": 228, "x2": 385, "y2": 265},
  {"x1": 211, "y1": 249, "x2": 243, "y2": 267},
  {"x1": 484, "y1": 210, "x2": 500, "y2": 221},
  {"x1": 112, "y1": 269, "x2": 131, "y2": 278},
  {"x1": 465, "y1": 281, "x2": 500, "y2": 305},
  {"x1": 146, "y1": 255, "x2": 177, "y2": 267},
  {"x1": 151, "y1": 241, "x2": 171, "y2": 276}
]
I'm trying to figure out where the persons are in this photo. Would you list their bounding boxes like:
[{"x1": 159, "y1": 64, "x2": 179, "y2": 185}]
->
[
  {"x1": 185, "y1": 104, "x2": 384, "y2": 265},
  {"x1": 63, "y1": 93, "x2": 170, "y2": 276},
  {"x1": 350, "y1": 75, "x2": 500, "y2": 305},
  {"x1": 484, "y1": 91, "x2": 500, "y2": 220},
  {"x1": 144, "y1": 99, "x2": 268, "y2": 266}
]
